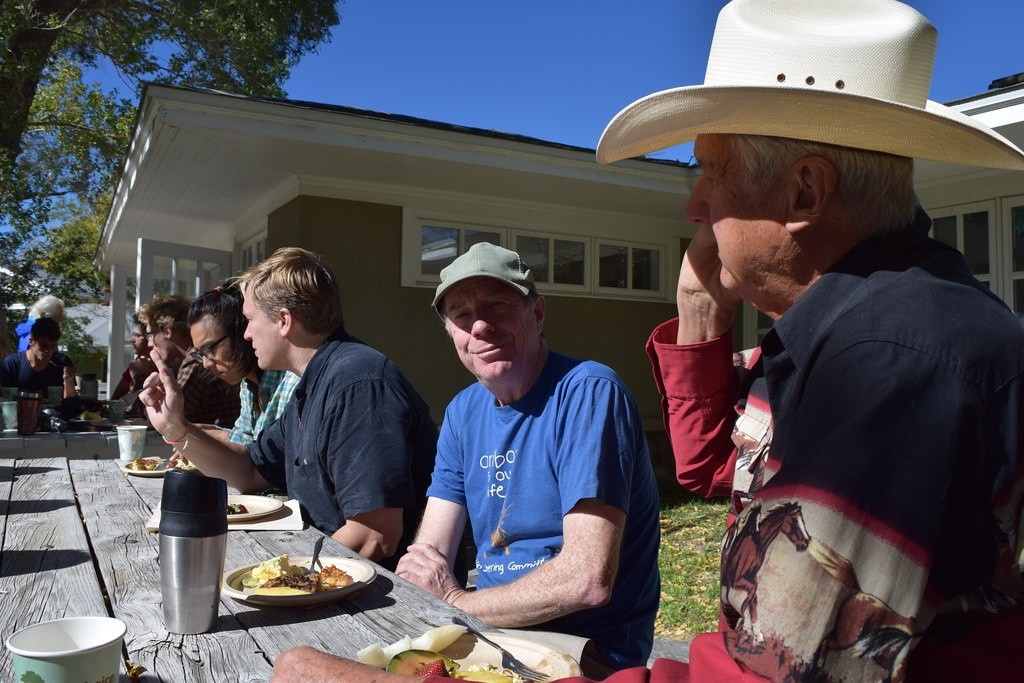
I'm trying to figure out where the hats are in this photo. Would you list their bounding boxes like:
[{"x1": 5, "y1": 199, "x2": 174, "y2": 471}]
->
[
  {"x1": 594, "y1": 1, "x2": 1024, "y2": 179},
  {"x1": 432, "y1": 241, "x2": 538, "y2": 323}
]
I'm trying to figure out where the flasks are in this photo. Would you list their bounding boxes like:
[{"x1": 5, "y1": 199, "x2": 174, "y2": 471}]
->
[{"x1": 157, "y1": 468, "x2": 228, "y2": 635}]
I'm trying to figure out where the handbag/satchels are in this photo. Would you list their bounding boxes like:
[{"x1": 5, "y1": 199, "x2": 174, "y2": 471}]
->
[{"x1": 36, "y1": 394, "x2": 103, "y2": 432}]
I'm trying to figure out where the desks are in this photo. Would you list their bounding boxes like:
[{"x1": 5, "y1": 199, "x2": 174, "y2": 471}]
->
[{"x1": 0, "y1": 417, "x2": 546, "y2": 683}]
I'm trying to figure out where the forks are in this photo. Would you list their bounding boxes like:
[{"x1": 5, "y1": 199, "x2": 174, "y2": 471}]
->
[
  {"x1": 111, "y1": 380, "x2": 163, "y2": 414},
  {"x1": 300, "y1": 536, "x2": 325, "y2": 587},
  {"x1": 48, "y1": 360, "x2": 64, "y2": 367},
  {"x1": 452, "y1": 617, "x2": 550, "y2": 682}
]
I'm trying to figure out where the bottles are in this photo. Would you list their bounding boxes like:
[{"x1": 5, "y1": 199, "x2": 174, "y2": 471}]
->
[{"x1": 80, "y1": 373, "x2": 98, "y2": 400}]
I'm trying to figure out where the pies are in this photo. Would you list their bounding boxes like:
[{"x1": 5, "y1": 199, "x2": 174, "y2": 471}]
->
[
  {"x1": 261, "y1": 564, "x2": 354, "y2": 594},
  {"x1": 131, "y1": 457, "x2": 160, "y2": 471}
]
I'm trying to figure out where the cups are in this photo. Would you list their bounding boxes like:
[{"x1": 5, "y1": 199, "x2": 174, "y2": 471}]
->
[
  {"x1": 1, "y1": 401, "x2": 18, "y2": 429},
  {"x1": 1, "y1": 387, "x2": 18, "y2": 402},
  {"x1": 16, "y1": 390, "x2": 40, "y2": 436},
  {"x1": 5, "y1": 615, "x2": 129, "y2": 683},
  {"x1": 116, "y1": 425, "x2": 148, "y2": 462},
  {"x1": 48, "y1": 386, "x2": 63, "y2": 403}
]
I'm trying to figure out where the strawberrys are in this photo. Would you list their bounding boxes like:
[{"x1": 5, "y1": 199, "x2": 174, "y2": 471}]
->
[
  {"x1": 417, "y1": 659, "x2": 451, "y2": 676},
  {"x1": 232, "y1": 504, "x2": 248, "y2": 513}
]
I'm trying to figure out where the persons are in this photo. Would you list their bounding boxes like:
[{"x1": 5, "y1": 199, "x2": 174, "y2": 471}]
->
[
  {"x1": 1, "y1": 294, "x2": 78, "y2": 403},
  {"x1": 272, "y1": 1, "x2": 1024, "y2": 683},
  {"x1": 110, "y1": 313, "x2": 181, "y2": 429},
  {"x1": 392, "y1": 244, "x2": 661, "y2": 681},
  {"x1": 140, "y1": 293, "x2": 242, "y2": 429},
  {"x1": 170, "y1": 284, "x2": 303, "y2": 465},
  {"x1": 138, "y1": 246, "x2": 470, "y2": 589}
]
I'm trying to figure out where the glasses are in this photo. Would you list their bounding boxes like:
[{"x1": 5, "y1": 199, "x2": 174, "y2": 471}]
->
[
  {"x1": 133, "y1": 332, "x2": 148, "y2": 341},
  {"x1": 142, "y1": 325, "x2": 166, "y2": 343},
  {"x1": 192, "y1": 328, "x2": 239, "y2": 363}
]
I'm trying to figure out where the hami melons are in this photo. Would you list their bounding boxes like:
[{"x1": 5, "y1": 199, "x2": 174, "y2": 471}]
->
[
  {"x1": 385, "y1": 648, "x2": 460, "y2": 674},
  {"x1": 450, "y1": 672, "x2": 513, "y2": 683}
]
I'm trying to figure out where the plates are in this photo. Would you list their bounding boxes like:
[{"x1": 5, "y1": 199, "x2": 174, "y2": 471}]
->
[
  {"x1": 70, "y1": 419, "x2": 107, "y2": 421},
  {"x1": 220, "y1": 555, "x2": 378, "y2": 607},
  {"x1": 158, "y1": 494, "x2": 284, "y2": 522},
  {"x1": 120, "y1": 459, "x2": 182, "y2": 477},
  {"x1": 440, "y1": 634, "x2": 584, "y2": 683}
]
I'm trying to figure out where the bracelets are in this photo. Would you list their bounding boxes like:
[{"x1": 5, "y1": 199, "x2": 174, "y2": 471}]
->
[
  {"x1": 162, "y1": 419, "x2": 200, "y2": 450},
  {"x1": 62, "y1": 381, "x2": 75, "y2": 386}
]
